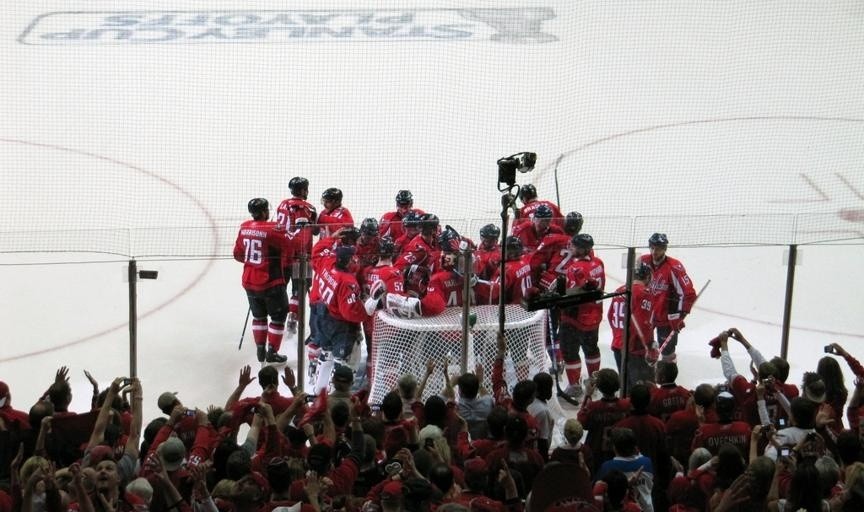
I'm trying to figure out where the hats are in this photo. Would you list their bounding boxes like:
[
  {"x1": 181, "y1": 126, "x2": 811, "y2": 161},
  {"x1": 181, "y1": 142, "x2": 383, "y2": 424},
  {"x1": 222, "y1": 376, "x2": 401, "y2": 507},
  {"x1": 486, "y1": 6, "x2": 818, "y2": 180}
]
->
[
  {"x1": 126, "y1": 477, "x2": 153, "y2": 504},
  {"x1": 334, "y1": 366, "x2": 353, "y2": 382},
  {"x1": 89, "y1": 445, "x2": 114, "y2": 463},
  {"x1": 597, "y1": 369, "x2": 620, "y2": 393},
  {"x1": 805, "y1": 380, "x2": 826, "y2": 403},
  {"x1": 158, "y1": 392, "x2": 178, "y2": 410},
  {"x1": 554, "y1": 418, "x2": 583, "y2": 450},
  {"x1": 159, "y1": 438, "x2": 185, "y2": 471}
]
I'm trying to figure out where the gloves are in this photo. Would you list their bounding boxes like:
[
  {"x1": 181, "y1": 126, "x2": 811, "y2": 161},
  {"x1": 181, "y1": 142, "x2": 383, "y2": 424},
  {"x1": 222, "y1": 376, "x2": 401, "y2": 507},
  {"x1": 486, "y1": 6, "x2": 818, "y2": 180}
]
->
[
  {"x1": 288, "y1": 200, "x2": 317, "y2": 224},
  {"x1": 644, "y1": 341, "x2": 659, "y2": 367},
  {"x1": 369, "y1": 279, "x2": 385, "y2": 300},
  {"x1": 668, "y1": 312, "x2": 685, "y2": 333},
  {"x1": 538, "y1": 271, "x2": 557, "y2": 292}
]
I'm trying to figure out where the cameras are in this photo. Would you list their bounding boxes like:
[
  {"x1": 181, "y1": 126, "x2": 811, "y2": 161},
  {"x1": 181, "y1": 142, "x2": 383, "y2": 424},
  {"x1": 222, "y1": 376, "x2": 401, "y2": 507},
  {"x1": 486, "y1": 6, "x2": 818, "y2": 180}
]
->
[
  {"x1": 372, "y1": 404, "x2": 384, "y2": 412},
  {"x1": 778, "y1": 446, "x2": 790, "y2": 462},
  {"x1": 305, "y1": 395, "x2": 316, "y2": 402},
  {"x1": 186, "y1": 409, "x2": 196, "y2": 417},
  {"x1": 726, "y1": 330, "x2": 732, "y2": 337},
  {"x1": 762, "y1": 378, "x2": 772, "y2": 385},
  {"x1": 760, "y1": 425, "x2": 769, "y2": 432},
  {"x1": 824, "y1": 345, "x2": 834, "y2": 353},
  {"x1": 777, "y1": 417, "x2": 787, "y2": 429}
]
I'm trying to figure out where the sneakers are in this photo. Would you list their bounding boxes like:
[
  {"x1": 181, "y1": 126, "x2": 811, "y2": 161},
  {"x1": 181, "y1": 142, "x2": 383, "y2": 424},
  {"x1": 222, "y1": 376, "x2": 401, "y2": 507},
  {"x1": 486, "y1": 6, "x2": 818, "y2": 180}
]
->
[
  {"x1": 286, "y1": 312, "x2": 297, "y2": 334},
  {"x1": 308, "y1": 351, "x2": 332, "y2": 377},
  {"x1": 563, "y1": 383, "x2": 583, "y2": 398},
  {"x1": 332, "y1": 360, "x2": 356, "y2": 374}
]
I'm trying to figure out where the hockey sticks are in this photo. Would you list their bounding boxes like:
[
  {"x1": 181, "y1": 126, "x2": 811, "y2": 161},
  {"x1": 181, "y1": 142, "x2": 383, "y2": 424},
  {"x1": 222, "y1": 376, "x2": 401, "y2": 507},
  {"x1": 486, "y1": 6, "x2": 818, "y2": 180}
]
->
[
  {"x1": 555, "y1": 152, "x2": 564, "y2": 213},
  {"x1": 549, "y1": 309, "x2": 580, "y2": 406}
]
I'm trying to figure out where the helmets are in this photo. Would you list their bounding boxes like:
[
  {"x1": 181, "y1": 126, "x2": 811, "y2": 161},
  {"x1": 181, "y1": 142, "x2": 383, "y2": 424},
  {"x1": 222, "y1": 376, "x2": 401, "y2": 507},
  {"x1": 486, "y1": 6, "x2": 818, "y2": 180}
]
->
[
  {"x1": 571, "y1": 234, "x2": 593, "y2": 249},
  {"x1": 518, "y1": 184, "x2": 537, "y2": 200},
  {"x1": 648, "y1": 233, "x2": 669, "y2": 249},
  {"x1": 403, "y1": 212, "x2": 460, "y2": 252},
  {"x1": 500, "y1": 236, "x2": 523, "y2": 250},
  {"x1": 248, "y1": 198, "x2": 268, "y2": 213},
  {"x1": 632, "y1": 261, "x2": 651, "y2": 277},
  {"x1": 534, "y1": 205, "x2": 553, "y2": 218},
  {"x1": 322, "y1": 188, "x2": 342, "y2": 203},
  {"x1": 565, "y1": 211, "x2": 583, "y2": 226},
  {"x1": 406, "y1": 270, "x2": 429, "y2": 296},
  {"x1": 337, "y1": 218, "x2": 395, "y2": 258},
  {"x1": 479, "y1": 224, "x2": 500, "y2": 240},
  {"x1": 396, "y1": 190, "x2": 413, "y2": 205},
  {"x1": 289, "y1": 177, "x2": 308, "y2": 194}
]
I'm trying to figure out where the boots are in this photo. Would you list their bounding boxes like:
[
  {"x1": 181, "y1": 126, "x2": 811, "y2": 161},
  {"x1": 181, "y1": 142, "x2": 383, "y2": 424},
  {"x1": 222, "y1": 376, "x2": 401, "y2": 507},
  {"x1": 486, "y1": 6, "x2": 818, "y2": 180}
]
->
[{"x1": 256, "y1": 343, "x2": 286, "y2": 363}]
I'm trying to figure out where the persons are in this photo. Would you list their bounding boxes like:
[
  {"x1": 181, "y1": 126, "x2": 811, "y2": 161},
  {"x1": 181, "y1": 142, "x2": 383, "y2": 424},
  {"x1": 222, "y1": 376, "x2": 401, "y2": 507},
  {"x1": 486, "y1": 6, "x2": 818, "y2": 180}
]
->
[
  {"x1": 233, "y1": 176, "x2": 697, "y2": 400},
  {"x1": 1, "y1": 329, "x2": 864, "y2": 512}
]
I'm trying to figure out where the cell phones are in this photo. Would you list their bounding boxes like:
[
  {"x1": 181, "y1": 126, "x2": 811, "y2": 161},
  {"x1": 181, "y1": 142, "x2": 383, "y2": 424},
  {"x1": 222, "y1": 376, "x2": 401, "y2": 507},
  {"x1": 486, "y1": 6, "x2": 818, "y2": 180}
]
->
[
  {"x1": 425, "y1": 437, "x2": 433, "y2": 451},
  {"x1": 123, "y1": 378, "x2": 133, "y2": 385}
]
